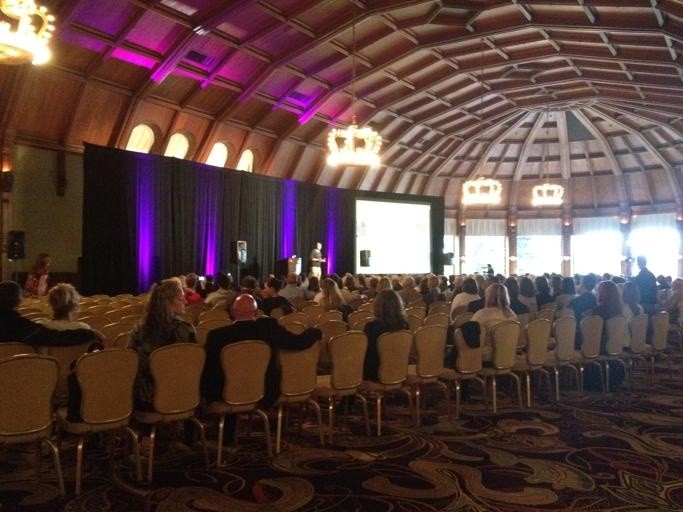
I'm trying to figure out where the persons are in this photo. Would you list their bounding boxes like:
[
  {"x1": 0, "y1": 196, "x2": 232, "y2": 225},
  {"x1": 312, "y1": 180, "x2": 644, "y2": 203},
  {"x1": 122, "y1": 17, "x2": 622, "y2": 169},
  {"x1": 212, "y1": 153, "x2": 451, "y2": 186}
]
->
[
  {"x1": 314, "y1": 253, "x2": 681, "y2": 415},
  {"x1": 307, "y1": 240, "x2": 325, "y2": 285},
  {"x1": 2, "y1": 251, "x2": 106, "y2": 407},
  {"x1": 126, "y1": 272, "x2": 319, "y2": 451}
]
